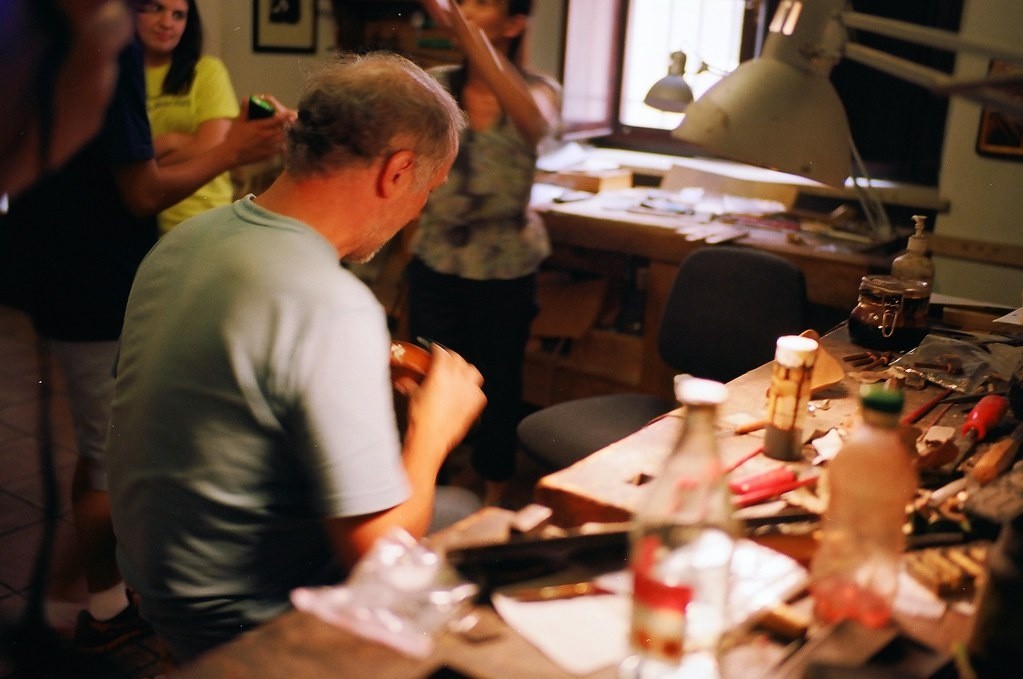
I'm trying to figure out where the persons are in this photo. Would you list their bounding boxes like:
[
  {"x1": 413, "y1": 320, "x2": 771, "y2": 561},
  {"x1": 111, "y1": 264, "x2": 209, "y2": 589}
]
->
[
  {"x1": 106, "y1": 49, "x2": 486, "y2": 652},
  {"x1": 1, "y1": 0, "x2": 290, "y2": 660},
  {"x1": 128, "y1": 0, "x2": 237, "y2": 231},
  {"x1": 375, "y1": 0, "x2": 564, "y2": 505}
]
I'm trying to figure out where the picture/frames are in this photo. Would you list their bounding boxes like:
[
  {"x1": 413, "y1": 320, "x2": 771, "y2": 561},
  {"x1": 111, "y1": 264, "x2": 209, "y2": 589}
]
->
[
  {"x1": 975, "y1": 57, "x2": 1023, "y2": 161},
  {"x1": 250, "y1": 0, "x2": 318, "y2": 55}
]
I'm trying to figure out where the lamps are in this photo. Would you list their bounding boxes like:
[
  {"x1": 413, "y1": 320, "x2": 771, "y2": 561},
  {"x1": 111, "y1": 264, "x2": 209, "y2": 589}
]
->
[
  {"x1": 671, "y1": 0, "x2": 1023, "y2": 190},
  {"x1": 645, "y1": 52, "x2": 897, "y2": 243}
]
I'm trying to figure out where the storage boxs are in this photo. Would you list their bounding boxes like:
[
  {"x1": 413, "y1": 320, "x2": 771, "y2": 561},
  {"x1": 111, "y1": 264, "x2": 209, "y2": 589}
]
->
[{"x1": 531, "y1": 275, "x2": 643, "y2": 403}]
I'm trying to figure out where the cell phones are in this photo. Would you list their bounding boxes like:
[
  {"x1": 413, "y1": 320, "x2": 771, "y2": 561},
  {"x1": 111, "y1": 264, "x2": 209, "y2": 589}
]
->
[{"x1": 247, "y1": 95, "x2": 275, "y2": 121}]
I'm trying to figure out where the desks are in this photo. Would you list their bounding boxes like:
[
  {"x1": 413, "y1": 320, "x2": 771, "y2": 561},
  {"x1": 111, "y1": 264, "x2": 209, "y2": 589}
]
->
[
  {"x1": 174, "y1": 303, "x2": 1023, "y2": 679},
  {"x1": 527, "y1": 163, "x2": 893, "y2": 406}
]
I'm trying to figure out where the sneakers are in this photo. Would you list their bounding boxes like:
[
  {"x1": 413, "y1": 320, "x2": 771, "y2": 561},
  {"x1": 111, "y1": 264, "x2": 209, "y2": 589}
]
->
[{"x1": 74, "y1": 589, "x2": 154, "y2": 653}]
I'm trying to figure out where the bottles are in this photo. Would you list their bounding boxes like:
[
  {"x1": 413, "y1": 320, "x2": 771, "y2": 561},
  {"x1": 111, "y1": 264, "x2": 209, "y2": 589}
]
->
[
  {"x1": 628, "y1": 377, "x2": 735, "y2": 679},
  {"x1": 810, "y1": 382, "x2": 916, "y2": 625},
  {"x1": 762, "y1": 334, "x2": 818, "y2": 463}
]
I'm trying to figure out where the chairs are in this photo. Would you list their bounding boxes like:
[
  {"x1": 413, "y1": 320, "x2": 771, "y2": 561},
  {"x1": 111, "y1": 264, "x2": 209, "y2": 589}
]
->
[{"x1": 514, "y1": 245, "x2": 804, "y2": 470}]
